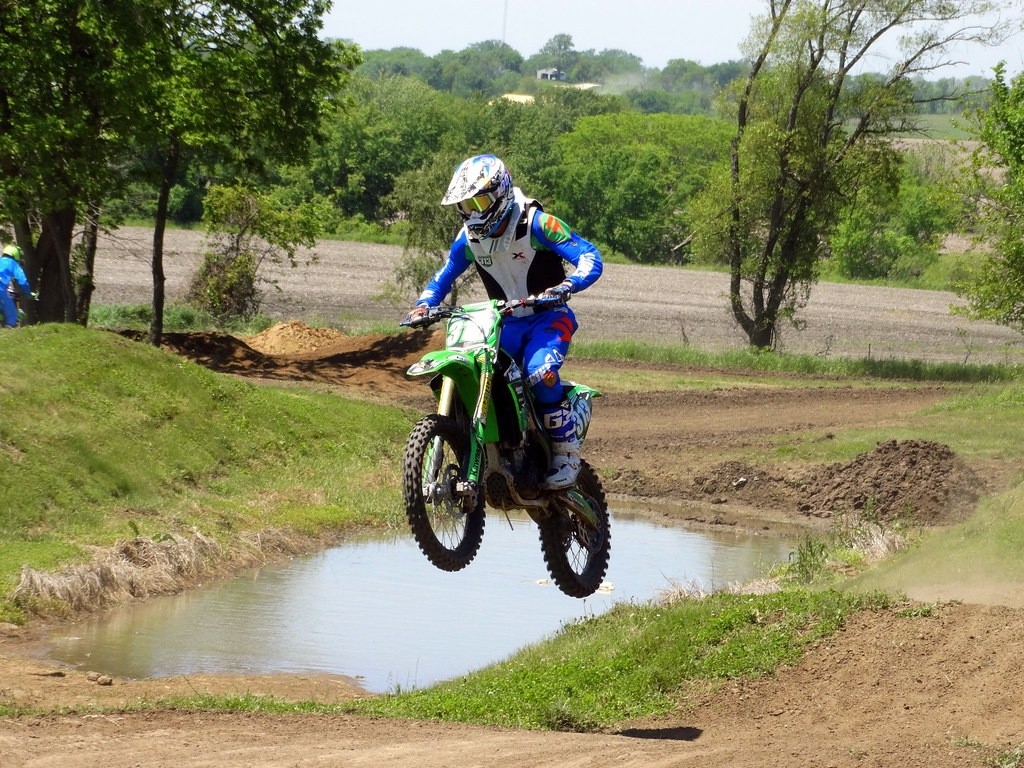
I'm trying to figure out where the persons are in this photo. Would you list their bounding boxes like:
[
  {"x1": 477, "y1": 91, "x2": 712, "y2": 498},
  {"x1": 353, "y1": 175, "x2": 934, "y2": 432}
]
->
[
  {"x1": 409, "y1": 153, "x2": 602, "y2": 494},
  {"x1": 0, "y1": 244, "x2": 33, "y2": 326}
]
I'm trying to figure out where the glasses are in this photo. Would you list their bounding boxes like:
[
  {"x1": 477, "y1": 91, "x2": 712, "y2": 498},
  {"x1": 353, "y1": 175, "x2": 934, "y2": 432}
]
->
[{"x1": 454, "y1": 189, "x2": 497, "y2": 216}]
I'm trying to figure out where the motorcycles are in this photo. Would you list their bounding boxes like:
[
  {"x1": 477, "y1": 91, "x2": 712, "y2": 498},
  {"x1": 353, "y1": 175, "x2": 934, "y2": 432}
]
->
[
  {"x1": 0, "y1": 288, "x2": 39, "y2": 329},
  {"x1": 399, "y1": 290, "x2": 612, "y2": 599}
]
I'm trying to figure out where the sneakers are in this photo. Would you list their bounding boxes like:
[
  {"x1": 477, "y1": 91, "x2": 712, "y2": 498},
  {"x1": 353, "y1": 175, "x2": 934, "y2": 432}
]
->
[{"x1": 547, "y1": 450, "x2": 582, "y2": 490}]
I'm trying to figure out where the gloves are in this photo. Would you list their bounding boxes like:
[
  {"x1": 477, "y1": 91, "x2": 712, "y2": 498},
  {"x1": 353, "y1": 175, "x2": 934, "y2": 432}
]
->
[
  {"x1": 404, "y1": 304, "x2": 430, "y2": 330},
  {"x1": 31, "y1": 292, "x2": 37, "y2": 301},
  {"x1": 544, "y1": 282, "x2": 571, "y2": 304}
]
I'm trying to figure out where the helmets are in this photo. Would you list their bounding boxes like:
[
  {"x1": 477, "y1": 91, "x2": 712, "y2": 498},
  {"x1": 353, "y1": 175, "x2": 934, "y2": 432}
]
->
[
  {"x1": 441, "y1": 154, "x2": 516, "y2": 240},
  {"x1": 3, "y1": 245, "x2": 20, "y2": 262}
]
